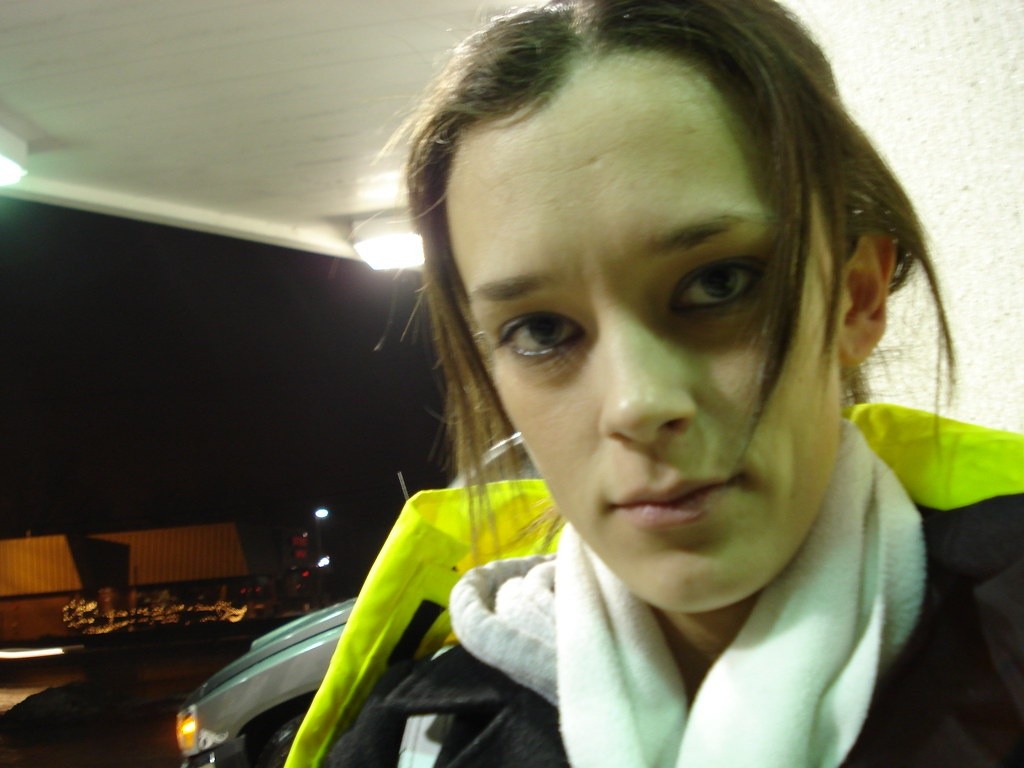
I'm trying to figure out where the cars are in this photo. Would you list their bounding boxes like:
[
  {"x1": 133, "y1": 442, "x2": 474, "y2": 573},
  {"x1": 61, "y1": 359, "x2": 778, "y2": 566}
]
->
[{"x1": 172, "y1": 594, "x2": 357, "y2": 768}]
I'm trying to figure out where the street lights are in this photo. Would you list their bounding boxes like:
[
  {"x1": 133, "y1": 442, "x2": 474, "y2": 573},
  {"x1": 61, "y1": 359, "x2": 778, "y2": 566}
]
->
[{"x1": 310, "y1": 505, "x2": 330, "y2": 607}]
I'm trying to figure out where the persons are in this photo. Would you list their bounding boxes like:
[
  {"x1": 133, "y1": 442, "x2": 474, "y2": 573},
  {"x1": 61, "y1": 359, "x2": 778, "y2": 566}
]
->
[{"x1": 269, "y1": 0, "x2": 1024, "y2": 768}]
